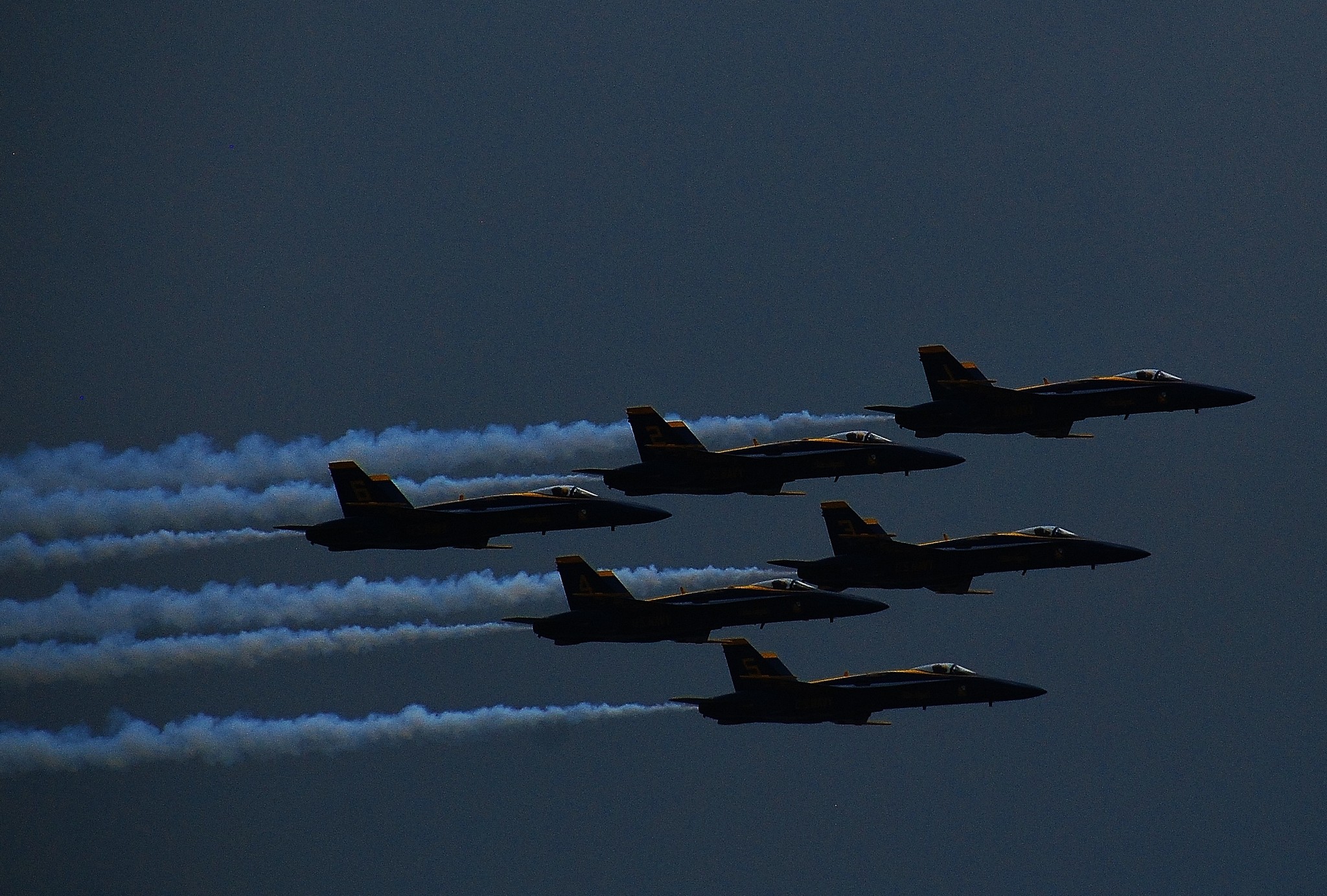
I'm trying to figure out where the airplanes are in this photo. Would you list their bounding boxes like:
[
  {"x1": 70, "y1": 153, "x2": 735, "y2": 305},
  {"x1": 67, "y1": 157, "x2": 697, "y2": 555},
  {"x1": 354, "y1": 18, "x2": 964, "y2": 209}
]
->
[
  {"x1": 764, "y1": 500, "x2": 1153, "y2": 594},
  {"x1": 862, "y1": 342, "x2": 1258, "y2": 441},
  {"x1": 271, "y1": 459, "x2": 676, "y2": 553},
  {"x1": 665, "y1": 638, "x2": 1048, "y2": 728},
  {"x1": 570, "y1": 404, "x2": 966, "y2": 491},
  {"x1": 499, "y1": 553, "x2": 891, "y2": 646}
]
